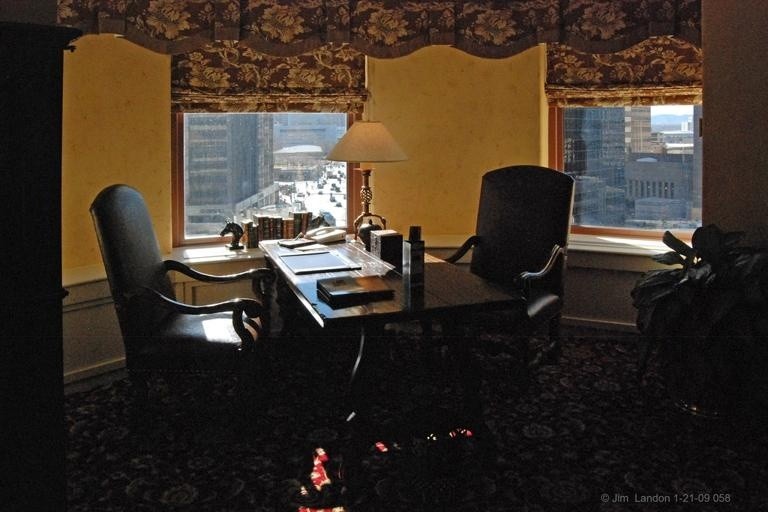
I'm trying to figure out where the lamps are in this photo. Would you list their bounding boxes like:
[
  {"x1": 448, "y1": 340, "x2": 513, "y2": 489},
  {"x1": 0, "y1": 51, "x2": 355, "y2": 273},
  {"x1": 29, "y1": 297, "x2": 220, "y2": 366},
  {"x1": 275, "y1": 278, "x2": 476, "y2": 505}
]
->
[{"x1": 323, "y1": 118, "x2": 409, "y2": 240}]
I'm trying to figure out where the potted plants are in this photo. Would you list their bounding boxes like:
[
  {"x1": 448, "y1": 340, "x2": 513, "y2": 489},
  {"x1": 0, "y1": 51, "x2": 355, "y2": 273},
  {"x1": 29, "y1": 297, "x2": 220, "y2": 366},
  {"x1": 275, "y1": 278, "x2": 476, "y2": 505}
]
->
[{"x1": 630, "y1": 220, "x2": 768, "y2": 473}]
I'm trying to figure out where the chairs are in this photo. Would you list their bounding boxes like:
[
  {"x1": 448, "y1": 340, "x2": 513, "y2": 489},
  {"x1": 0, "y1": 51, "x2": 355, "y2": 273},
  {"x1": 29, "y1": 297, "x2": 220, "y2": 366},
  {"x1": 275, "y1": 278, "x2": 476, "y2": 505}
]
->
[
  {"x1": 435, "y1": 165, "x2": 578, "y2": 402},
  {"x1": 86, "y1": 183, "x2": 281, "y2": 446}
]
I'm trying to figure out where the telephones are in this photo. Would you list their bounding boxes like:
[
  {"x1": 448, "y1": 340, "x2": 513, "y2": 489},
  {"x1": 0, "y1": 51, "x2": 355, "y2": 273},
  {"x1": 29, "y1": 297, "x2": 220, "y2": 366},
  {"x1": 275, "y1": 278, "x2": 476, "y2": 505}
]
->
[{"x1": 303, "y1": 225, "x2": 347, "y2": 244}]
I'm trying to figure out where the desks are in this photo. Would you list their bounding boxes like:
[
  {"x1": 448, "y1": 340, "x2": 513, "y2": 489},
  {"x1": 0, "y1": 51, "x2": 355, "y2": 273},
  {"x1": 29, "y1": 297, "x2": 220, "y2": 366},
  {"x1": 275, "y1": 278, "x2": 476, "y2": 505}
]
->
[{"x1": 257, "y1": 234, "x2": 529, "y2": 494}]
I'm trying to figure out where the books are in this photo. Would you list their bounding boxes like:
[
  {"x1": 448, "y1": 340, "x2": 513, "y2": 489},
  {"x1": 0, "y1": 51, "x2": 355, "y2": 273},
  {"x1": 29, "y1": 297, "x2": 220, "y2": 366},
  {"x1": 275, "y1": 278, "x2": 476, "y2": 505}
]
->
[{"x1": 241, "y1": 210, "x2": 313, "y2": 248}]
